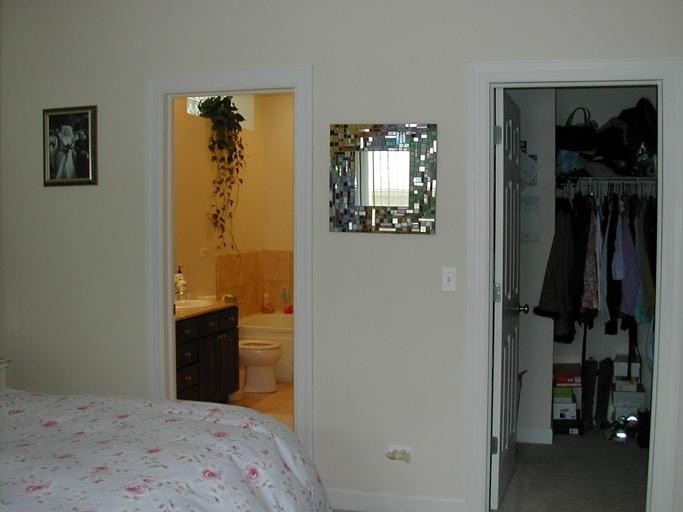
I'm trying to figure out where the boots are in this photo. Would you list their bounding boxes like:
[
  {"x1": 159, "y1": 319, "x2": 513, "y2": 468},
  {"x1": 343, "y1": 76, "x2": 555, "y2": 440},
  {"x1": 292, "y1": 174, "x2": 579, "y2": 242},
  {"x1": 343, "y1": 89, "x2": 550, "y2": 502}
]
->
[
  {"x1": 580, "y1": 360, "x2": 597, "y2": 434},
  {"x1": 596, "y1": 360, "x2": 613, "y2": 430}
]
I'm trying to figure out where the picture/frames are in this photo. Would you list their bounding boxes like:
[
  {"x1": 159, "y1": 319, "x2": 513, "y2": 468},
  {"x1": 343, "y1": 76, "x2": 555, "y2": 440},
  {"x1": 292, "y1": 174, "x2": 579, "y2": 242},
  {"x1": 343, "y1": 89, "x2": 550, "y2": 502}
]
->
[{"x1": 41, "y1": 105, "x2": 98, "y2": 187}]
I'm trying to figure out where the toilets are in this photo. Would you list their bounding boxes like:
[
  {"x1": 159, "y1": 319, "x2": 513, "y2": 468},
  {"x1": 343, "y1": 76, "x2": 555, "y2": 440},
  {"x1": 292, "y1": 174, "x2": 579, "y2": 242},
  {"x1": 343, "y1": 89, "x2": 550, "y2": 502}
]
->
[{"x1": 239, "y1": 339, "x2": 282, "y2": 393}]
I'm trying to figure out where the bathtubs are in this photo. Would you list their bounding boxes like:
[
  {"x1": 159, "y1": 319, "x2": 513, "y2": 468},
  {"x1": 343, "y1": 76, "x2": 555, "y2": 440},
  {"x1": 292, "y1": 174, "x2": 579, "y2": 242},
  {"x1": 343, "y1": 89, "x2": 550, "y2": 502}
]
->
[{"x1": 238, "y1": 311, "x2": 294, "y2": 382}]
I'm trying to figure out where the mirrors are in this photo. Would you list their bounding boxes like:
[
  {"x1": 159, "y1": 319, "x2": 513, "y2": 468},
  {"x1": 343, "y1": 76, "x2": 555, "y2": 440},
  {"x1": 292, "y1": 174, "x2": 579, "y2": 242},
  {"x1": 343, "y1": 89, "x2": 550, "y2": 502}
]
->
[{"x1": 327, "y1": 122, "x2": 438, "y2": 234}]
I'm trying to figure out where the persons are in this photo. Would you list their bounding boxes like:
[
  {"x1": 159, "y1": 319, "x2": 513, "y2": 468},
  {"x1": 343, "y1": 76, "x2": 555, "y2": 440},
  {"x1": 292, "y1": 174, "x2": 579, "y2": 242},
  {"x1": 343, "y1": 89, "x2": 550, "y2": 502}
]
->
[{"x1": 49, "y1": 125, "x2": 90, "y2": 180}]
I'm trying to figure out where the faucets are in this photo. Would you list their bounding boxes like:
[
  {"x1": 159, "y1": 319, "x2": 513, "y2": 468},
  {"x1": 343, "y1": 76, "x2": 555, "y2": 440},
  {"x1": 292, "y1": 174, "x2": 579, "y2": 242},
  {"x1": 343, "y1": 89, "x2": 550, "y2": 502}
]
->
[{"x1": 175, "y1": 289, "x2": 190, "y2": 300}]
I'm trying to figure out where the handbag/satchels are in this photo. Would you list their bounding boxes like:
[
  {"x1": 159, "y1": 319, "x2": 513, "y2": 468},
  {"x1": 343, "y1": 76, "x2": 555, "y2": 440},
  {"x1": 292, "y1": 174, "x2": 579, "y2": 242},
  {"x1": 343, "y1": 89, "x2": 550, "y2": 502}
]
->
[
  {"x1": 599, "y1": 97, "x2": 657, "y2": 175},
  {"x1": 556, "y1": 120, "x2": 598, "y2": 176}
]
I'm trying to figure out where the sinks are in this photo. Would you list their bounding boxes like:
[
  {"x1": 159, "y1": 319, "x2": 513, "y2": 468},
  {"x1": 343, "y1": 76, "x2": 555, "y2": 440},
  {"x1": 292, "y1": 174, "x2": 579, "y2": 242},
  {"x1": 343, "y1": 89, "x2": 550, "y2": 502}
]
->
[{"x1": 175, "y1": 299, "x2": 215, "y2": 308}]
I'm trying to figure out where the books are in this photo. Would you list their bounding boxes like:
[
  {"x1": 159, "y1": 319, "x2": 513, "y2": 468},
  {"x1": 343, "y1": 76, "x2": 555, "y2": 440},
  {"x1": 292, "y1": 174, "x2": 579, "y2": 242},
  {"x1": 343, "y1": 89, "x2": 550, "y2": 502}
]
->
[{"x1": 555, "y1": 367, "x2": 582, "y2": 387}]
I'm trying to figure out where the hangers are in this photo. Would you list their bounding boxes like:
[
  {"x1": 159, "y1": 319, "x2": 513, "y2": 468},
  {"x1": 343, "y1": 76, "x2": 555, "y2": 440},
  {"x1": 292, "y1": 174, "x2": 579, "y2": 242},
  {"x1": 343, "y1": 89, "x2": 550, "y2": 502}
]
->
[{"x1": 562, "y1": 178, "x2": 656, "y2": 213}]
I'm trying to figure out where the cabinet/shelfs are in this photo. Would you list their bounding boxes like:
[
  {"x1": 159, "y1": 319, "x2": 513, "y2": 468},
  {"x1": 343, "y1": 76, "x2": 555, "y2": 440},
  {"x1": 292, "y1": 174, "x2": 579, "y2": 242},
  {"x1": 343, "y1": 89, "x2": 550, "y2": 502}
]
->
[{"x1": 174, "y1": 304, "x2": 240, "y2": 402}]
